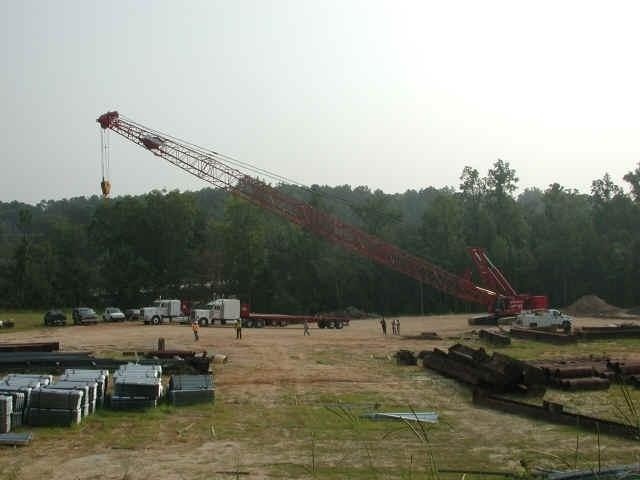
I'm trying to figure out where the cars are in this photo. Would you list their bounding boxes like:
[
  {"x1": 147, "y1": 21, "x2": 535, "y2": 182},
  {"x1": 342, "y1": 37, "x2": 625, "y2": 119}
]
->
[
  {"x1": 101, "y1": 307, "x2": 124, "y2": 322},
  {"x1": 44, "y1": 311, "x2": 66, "y2": 327},
  {"x1": 126, "y1": 310, "x2": 140, "y2": 322}
]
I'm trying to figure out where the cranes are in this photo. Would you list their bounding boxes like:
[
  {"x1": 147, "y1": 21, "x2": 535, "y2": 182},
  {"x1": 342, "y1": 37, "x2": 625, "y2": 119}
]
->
[{"x1": 98, "y1": 111, "x2": 546, "y2": 324}]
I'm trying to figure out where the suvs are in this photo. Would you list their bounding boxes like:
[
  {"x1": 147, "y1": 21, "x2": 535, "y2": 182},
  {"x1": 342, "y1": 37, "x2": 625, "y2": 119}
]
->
[{"x1": 73, "y1": 307, "x2": 99, "y2": 325}]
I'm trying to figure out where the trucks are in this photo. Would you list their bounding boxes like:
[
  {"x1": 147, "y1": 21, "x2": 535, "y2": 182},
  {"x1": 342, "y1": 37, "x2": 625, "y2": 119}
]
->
[
  {"x1": 191, "y1": 297, "x2": 350, "y2": 329},
  {"x1": 138, "y1": 299, "x2": 194, "y2": 325}
]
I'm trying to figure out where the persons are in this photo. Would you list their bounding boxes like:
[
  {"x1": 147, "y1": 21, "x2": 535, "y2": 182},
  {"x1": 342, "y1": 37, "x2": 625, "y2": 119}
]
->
[
  {"x1": 380, "y1": 317, "x2": 387, "y2": 336},
  {"x1": 234, "y1": 318, "x2": 242, "y2": 340},
  {"x1": 391, "y1": 319, "x2": 397, "y2": 336},
  {"x1": 191, "y1": 321, "x2": 200, "y2": 341},
  {"x1": 396, "y1": 320, "x2": 400, "y2": 336},
  {"x1": 303, "y1": 320, "x2": 310, "y2": 337}
]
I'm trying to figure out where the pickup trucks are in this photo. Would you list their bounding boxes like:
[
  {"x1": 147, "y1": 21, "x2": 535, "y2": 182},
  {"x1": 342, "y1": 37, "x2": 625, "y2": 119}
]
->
[{"x1": 521, "y1": 309, "x2": 572, "y2": 333}]
